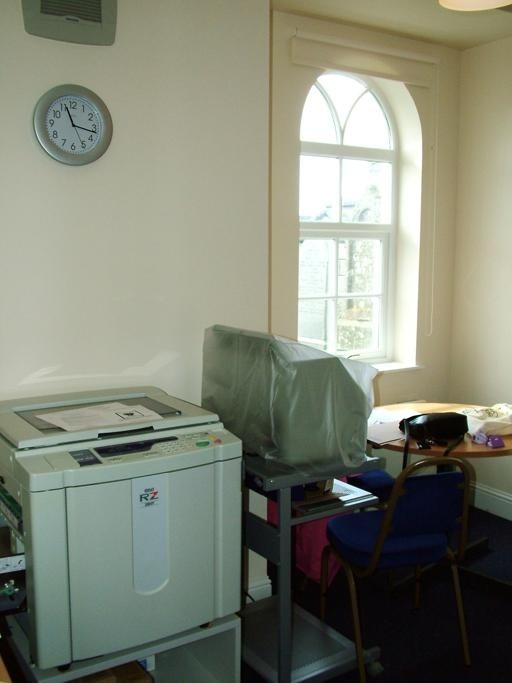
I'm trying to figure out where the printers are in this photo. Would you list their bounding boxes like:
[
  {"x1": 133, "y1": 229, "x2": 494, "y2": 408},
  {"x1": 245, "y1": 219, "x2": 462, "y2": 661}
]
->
[{"x1": 0, "y1": 388, "x2": 242, "y2": 672}]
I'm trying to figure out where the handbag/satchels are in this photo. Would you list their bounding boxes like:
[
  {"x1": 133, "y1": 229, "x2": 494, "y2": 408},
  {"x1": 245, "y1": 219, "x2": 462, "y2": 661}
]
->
[
  {"x1": 459, "y1": 403, "x2": 512, "y2": 437},
  {"x1": 400, "y1": 412, "x2": 468, "y2": 442}
]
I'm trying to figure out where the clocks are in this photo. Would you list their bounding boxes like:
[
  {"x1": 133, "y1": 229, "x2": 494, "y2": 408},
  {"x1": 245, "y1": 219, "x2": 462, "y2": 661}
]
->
[{"x1": 33, "y1": 83, "x2": 114, "y2": 167}]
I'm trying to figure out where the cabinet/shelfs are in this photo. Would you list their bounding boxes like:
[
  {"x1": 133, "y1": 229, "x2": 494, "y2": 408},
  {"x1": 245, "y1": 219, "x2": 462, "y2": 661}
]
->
[{"x1": 241, "y1": 453, "x2": 387, "y2": 683}]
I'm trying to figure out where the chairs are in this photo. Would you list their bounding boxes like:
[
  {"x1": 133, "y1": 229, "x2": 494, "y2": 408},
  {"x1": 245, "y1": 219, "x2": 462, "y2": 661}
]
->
[{"x1": 320, "y1": 456, "x2": 470, "y2": 682}]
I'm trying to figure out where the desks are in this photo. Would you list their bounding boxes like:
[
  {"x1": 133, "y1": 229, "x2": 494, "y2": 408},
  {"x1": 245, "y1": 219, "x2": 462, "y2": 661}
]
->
[{"x1": 368, "y1": 403, "x2": 511, "y2": 597}]
